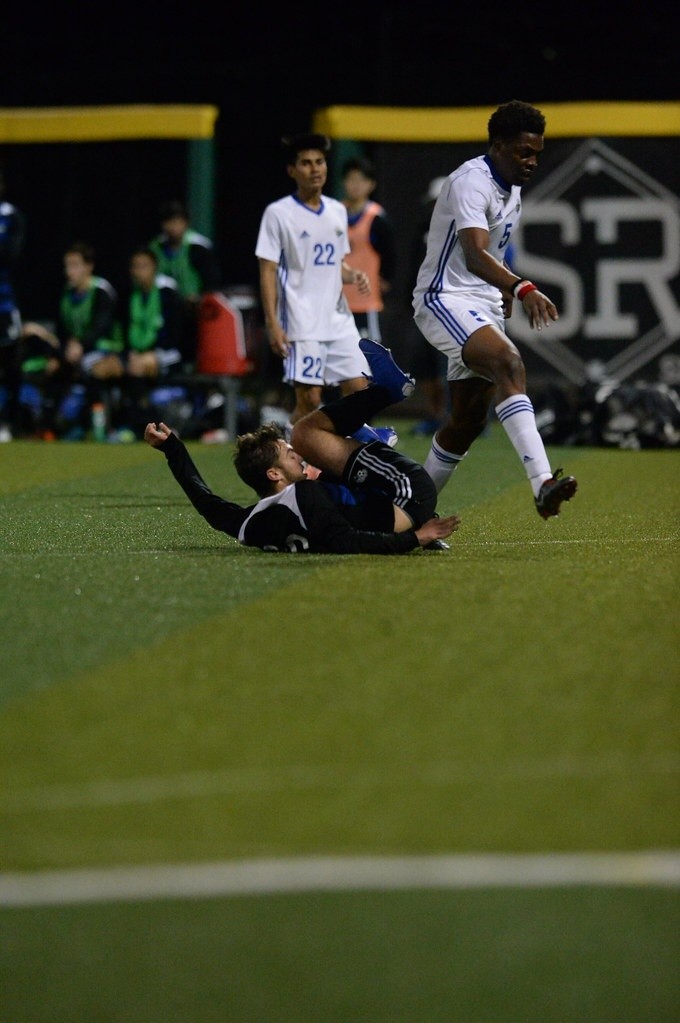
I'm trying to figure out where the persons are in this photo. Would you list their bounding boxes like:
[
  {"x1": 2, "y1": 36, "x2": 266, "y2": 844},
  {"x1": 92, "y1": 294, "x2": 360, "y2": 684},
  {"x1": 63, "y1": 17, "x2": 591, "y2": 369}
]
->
[
  {"x1": 409, "y1": 102, "x2": 581, "y2": 520},
  {"x1": 146, "y1": 341, "x2": 462, "y2": 553},
  {"x1": 256, "y1": 138, "x2": 373, "y2": 441},
  {"x1": 339, "y1": 158, "x2": 404, "y2": 346},
  {"x1": 0, "y1": 204, "x2": 293, "y2": 445}
]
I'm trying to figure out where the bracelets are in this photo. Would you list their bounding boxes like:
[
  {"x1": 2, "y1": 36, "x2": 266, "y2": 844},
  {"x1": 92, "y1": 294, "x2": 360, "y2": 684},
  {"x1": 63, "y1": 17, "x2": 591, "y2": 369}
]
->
[{"x1": 510, "y1": 277, "x2": 537, "y2": 301}]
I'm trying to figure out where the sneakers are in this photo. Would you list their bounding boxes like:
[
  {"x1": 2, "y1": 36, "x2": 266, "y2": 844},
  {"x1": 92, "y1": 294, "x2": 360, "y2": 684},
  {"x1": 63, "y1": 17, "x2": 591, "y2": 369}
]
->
[
  {"x1": 534, "y1": 467, "x2": 577, "y2": 521},
  {"x1": 360, "y1": 336, "x2": 416, "y2": 401},
  {"x1": 422, "y1": 514, "x2": 451, "y2": 550},
  {"x1": 349, "y1": 423, "x2": 399, "y2": 448}
]
